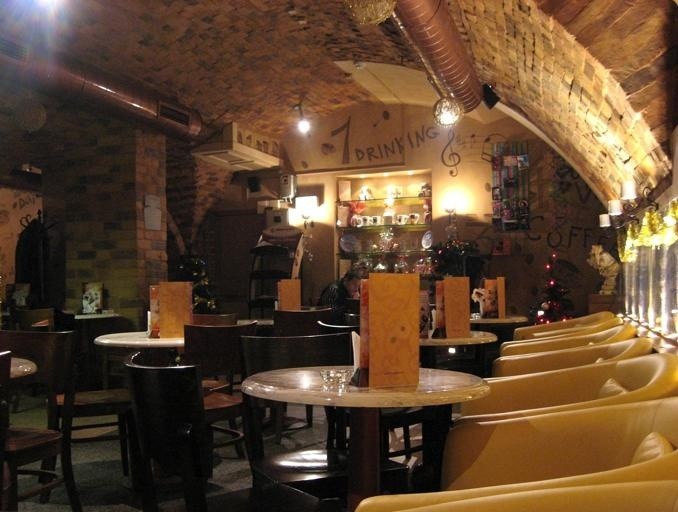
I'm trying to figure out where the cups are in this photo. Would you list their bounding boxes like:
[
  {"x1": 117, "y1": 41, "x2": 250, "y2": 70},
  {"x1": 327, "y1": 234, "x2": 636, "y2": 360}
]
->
[
  {"x1": 360, "y1": 214, "x2": 384, "y2": 225},
  {"x1": 397, "y1": 212, "x2": 420, "y2": 226}
]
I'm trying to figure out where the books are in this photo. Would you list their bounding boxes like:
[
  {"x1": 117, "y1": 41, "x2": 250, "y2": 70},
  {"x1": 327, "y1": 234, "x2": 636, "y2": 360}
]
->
[
  {"x1": 483, "y1": 277, "x2": 505, "y2": 318},
  {"x1": 348, "y1": 272, "x2": 420, "y2": 388},
  {"x1": 277, "y1": 278, "x2": 302, "y2": 312},
  {"x1": 419, "y1": 290, "x2": 429, "y2": 339},
  {"x1": 432, "y1": 276, "x2": 471, "y2": 338},
  {"x1": 147, "y1": 281, "x2": 194, "y2": 337},
  {"x1": 80, "y1": 282, "x2": 104, "y2": 313}
]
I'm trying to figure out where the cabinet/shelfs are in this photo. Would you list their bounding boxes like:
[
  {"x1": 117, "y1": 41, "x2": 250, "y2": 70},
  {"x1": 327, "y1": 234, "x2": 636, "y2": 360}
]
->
[
  {"x1": 247, "y1": 245, "x2": 292, "y2": 319},
  {"x1": 588, "y1": 293, "x2": 624, "y2": 316}
]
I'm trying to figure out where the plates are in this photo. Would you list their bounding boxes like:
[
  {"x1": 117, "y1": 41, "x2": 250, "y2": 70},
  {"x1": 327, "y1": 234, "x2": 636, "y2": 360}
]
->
[
  {"x1": 420, "y1": 230, "x2": 433, "y2": 250},
  {"x1": 339, "y1": 233, "x2": 360, "y2": 254}
]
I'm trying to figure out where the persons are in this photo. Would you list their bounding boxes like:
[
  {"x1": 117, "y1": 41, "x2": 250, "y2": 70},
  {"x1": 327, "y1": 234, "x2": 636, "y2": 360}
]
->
[{"x1": 318, "y1": 269, "x2": 361, "y2": 310}]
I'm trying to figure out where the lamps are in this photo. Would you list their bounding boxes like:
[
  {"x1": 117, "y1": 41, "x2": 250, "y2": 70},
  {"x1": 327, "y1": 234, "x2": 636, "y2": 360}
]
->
[
  {"x1": 298, "y1": 104, "x2": 312, "y2": 134},
  {"x1": 597, "y1": 179, "x2": 638, "y2": 228}
]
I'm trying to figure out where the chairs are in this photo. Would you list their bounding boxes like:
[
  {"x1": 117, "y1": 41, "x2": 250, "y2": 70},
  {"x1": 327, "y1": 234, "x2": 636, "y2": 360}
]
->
[{"x1": 0, "y1": 304, "x2": 678, "y2": 512}]
